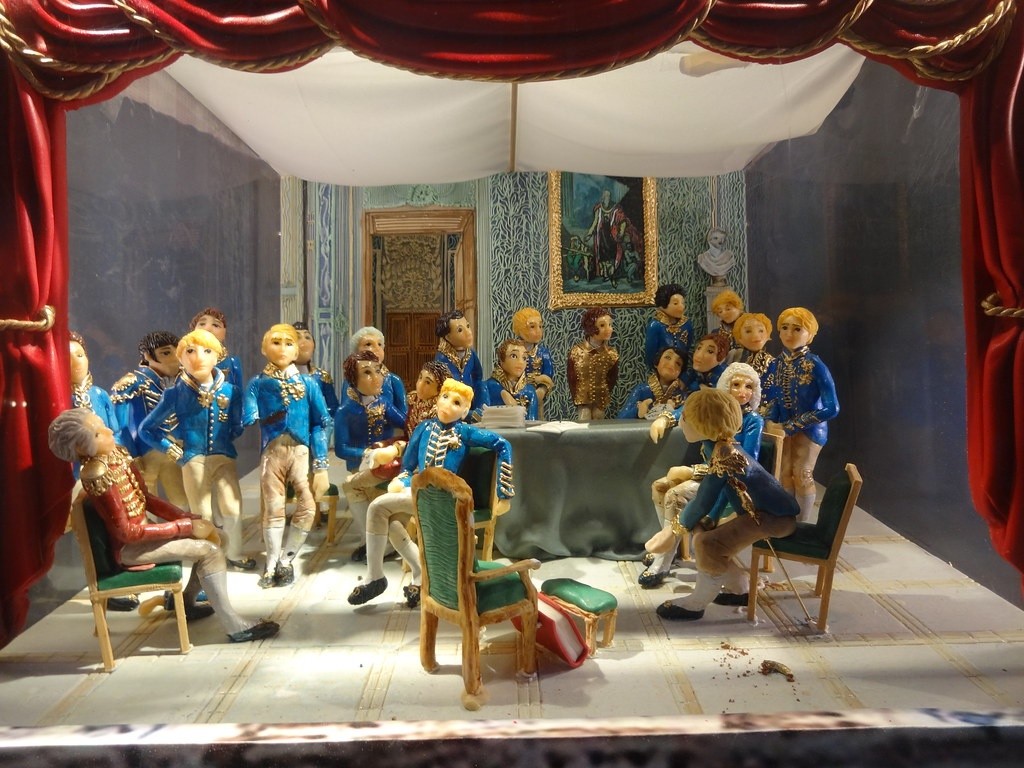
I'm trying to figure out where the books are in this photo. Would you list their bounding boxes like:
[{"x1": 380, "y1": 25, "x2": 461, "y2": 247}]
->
[{"x1": 509, "y1": 592, "x2": 589, "y2": 668}]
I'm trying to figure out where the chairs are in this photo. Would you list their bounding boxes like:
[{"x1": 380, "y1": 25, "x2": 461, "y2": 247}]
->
[
  {"x1": 746, "y1": 464, "x2": 865, "y2": 634},
  {"x1": 676, "y1": 431, "x2": 785, "y2": 574},
  {"x1": 68, "y1": 490, "x2": 192, "y2": 675},
  {"x1": 410, "y1": 466, "x2": 543, "y2": 698},
  {"x1": 259, "y1": 459, "x2": 340, "y2": 549},
  {"x1": 401, "y1": 446, "x2": 503, "y2": 577}
]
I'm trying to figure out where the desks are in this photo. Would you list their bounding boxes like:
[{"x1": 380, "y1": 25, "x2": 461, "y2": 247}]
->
[{"x1": 469, "y1": 416, "x2": 691, "y2": 560}]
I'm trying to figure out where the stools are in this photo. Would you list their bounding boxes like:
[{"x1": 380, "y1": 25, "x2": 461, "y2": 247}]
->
[{"x1": 539, "y1": 577, "x2": 620, "y2": 659}]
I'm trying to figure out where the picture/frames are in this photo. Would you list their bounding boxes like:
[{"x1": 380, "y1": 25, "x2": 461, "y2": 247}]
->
[{"x1": 547, "y1": 170, "x2": 660, "y2": 311}]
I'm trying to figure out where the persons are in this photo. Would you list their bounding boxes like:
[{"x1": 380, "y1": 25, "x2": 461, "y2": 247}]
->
[
  {"x1": 340, "y1": 325, "x2": 407, "y2": 415},
  {"x1": 638, "y1": 361, "x2": 765, "y2": 587},
  {"x1": 697, "y1": 227, "x2": 736, "y2": 282},
  {"x1": 62, "y1": 330, "x2": 124, "y2": 531},
  {"x1": 241, "y1": 322, "x2": 332, "y2": 587},
  {"x1": 567, "y1": 307, "x2": 620, "y2": 421},
  {"x1": 686, "y1": 332, "x2": 730, "y2": 393},
  {"x1": 760, "y1": 306, "x2": 840, "y2": 523},
  {"x1": 291, "y1": 322, "x2": 340, "y2": 437},
  {"x1": 346, "y1": 379, "x2": 515, "y2": 606},
  {"x1": 191, "y1": 308, "x2": 243, "y2": 391},
  {"x1": 644, "y1": 283, "x2": 692, "y2": 384},
  {"x1": 433, "y1": 309, "x2": 483, "y2": 424},
  {"x1": 404, "y1": 360, "x2": 453, "y2": 446},
  {"x1": 616, "y1": 345, "x2": 693, "y2": 445},
  {"x1": 49, "y1": 406, "x2": 280, "y2": 643},
  {"x1": 480, "y1": 337, "x2": 538, "y2": 421},
  {"x1": 645, "y1": 387, "x2": 801, "y2": 623},
  {"x1": 732, "y1": 312, "x2": 778, "y2": 425},
  {"x1": 333, "y1": 350, "x2": 408, "y2": 562},
  {"x1": 512, "y1": 306, "x2": 554, "y2": 420},
  {"x1": 709, "y1": 288, "x2": 746, "y2": 350},
  {"x1": 136, "y1": 329, "x2": 257, "y2": 572},
  {"x1": 108, "y1": 331, "x2": 189, "y2": 521}
]
